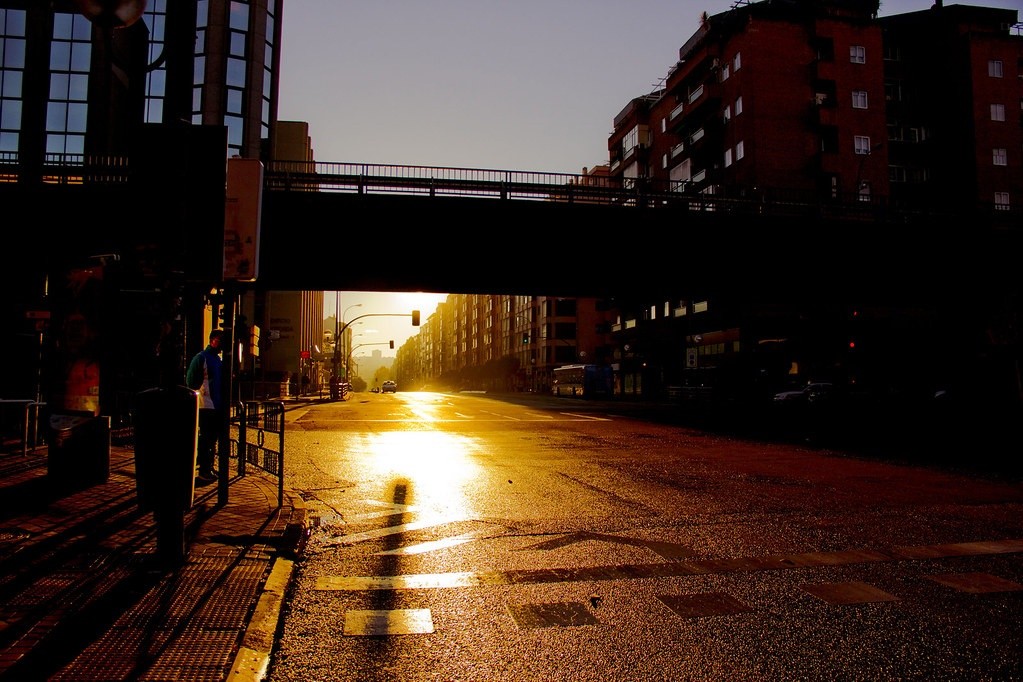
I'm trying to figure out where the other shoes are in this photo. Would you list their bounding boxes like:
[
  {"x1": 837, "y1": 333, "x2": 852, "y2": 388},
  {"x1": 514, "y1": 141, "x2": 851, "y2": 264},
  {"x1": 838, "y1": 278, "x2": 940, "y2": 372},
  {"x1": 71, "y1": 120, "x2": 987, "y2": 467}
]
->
[
  {"x1": 211, "y1": 469, "x2": 219, "y2": 479},
  {"x1": 199, "y1": 470, "x2": 218, "y2": 481}
]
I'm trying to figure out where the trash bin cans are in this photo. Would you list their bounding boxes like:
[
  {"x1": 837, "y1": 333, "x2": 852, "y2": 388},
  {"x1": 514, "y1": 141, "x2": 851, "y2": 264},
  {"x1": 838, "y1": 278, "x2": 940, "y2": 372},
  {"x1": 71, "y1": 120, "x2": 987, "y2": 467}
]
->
[{"x1": 45, "y1": 409, "x2": 112, "y2": 487}]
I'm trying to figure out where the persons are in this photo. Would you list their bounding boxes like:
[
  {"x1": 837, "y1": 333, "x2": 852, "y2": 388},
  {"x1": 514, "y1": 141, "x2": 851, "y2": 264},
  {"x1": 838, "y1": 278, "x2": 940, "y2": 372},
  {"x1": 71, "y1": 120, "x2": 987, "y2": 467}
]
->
[
  {"x1": 301, "y1": 373, "x2": 310, "y2": 395},
  {"x1": 187, "y1": 330, "x2": 223, "y2": 480}
]
